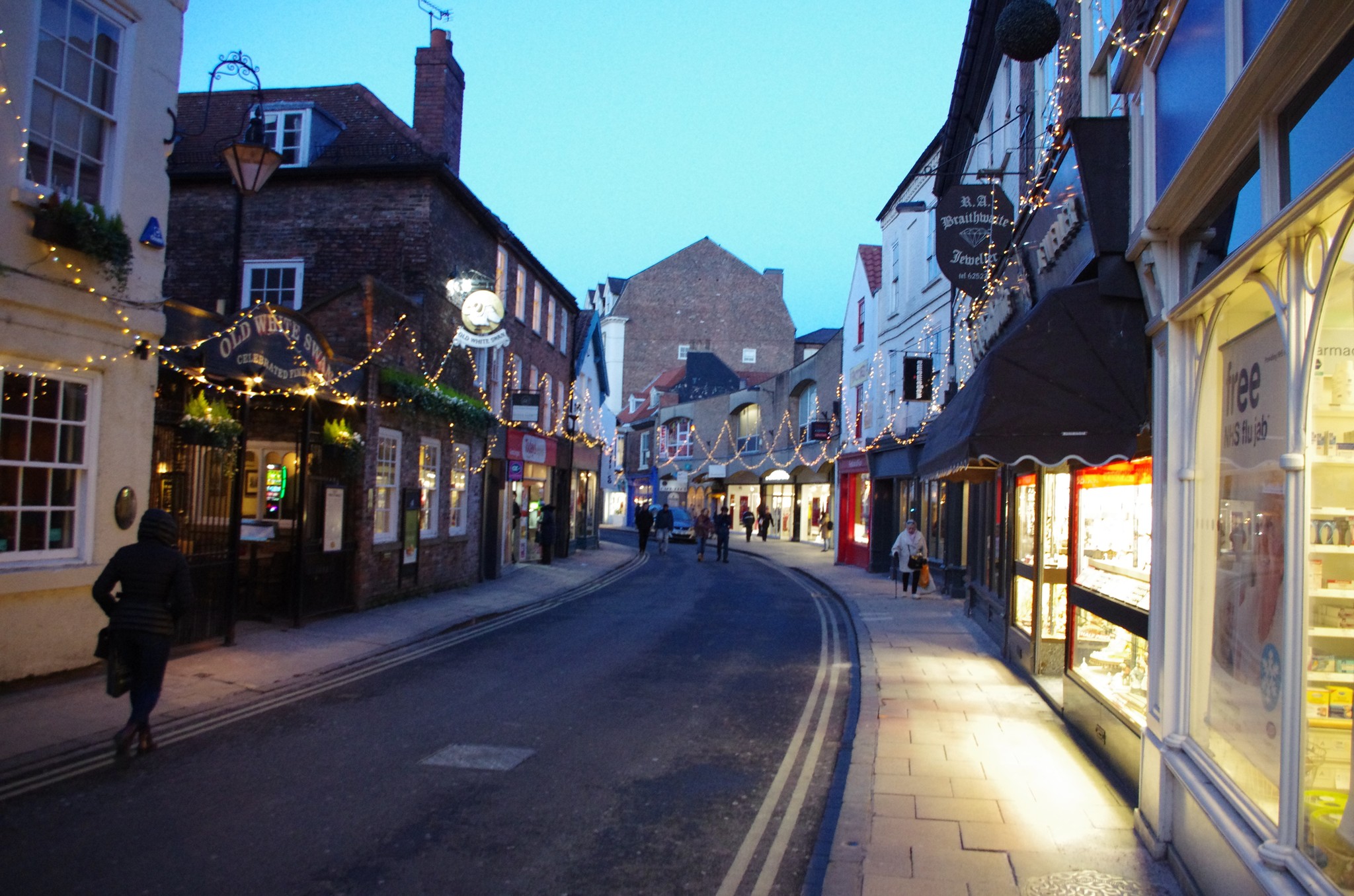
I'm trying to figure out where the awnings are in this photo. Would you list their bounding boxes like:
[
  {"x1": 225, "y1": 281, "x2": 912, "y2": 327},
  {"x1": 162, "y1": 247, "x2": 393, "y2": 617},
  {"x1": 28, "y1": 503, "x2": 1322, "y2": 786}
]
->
[{"x1": 915, "y1": 279, "x2": 1152, "y2": 485}]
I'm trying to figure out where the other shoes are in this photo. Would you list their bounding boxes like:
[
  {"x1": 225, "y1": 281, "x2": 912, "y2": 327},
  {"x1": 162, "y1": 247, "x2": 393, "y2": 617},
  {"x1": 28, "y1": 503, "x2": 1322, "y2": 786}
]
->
[
  {"x1": 717, "y1": 555, "x2": 721, "y2": 562},
  {"x1": 115, "y1": 729, "x2": 133, "y2": 763},
  {"x1": 912, "y1": 594, "x2": 921, "y2": 599},
  {"x1": 902, "y1": 592, "x2": 907, "y2": 597},
  {"x1": 723, "y1": 559, "x2": 729, "y2": 563},
  {"x1": 137, "y1": 738, "x2": 158, "y2": 755}
]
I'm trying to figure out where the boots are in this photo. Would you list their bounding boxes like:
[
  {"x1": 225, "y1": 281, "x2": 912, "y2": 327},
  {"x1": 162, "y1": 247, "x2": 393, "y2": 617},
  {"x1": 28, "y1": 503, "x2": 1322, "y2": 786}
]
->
[{"x1": 698, "y1": 553, "x2": 704, "y2": 561}]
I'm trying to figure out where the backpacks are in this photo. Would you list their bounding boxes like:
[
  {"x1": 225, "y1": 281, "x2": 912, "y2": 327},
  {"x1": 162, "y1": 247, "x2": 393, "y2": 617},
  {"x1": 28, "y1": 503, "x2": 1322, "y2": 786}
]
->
[{"x1": 827, "y1": 522, "x2": 833, "y2": 530}]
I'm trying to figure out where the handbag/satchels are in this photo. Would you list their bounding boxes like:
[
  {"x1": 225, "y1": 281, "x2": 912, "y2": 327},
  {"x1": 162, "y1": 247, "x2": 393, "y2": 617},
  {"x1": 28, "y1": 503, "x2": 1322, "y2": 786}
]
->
[
  {"x1": 917, "y1": 564, "x2": 935, "y2": 596},
  {"x1": 908, "y1": 556, "x2": 923, "y2": 569}
]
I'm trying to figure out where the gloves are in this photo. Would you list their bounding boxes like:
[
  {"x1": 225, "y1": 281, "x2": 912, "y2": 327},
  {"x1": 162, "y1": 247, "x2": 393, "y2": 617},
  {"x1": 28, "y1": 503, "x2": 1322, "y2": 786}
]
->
[{"x1": 895, "y1": 552, "x2": 899, "y2": 558}]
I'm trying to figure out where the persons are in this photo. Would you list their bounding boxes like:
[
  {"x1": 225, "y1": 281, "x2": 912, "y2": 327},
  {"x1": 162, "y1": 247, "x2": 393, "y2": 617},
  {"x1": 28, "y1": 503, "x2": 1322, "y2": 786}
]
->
[
  {"x1": 635, "y1": 503, "x2": 641, "y2": 517},
  {"x1": 538, "y1": 504, "x2": 556, "y2": 566},
  {"x1": 759, "y1": 506, "x2": 774, "y2": 542},
  {"x1": 635, "y1": 503, "x2": 654, "y2": 553},
  {"x1": 654, "y1": 504, "x2": 674, "y2": 555},
  {"x1": 93, "y1": 508, "x2": 189, "y2": 767},
  {"x1": 690, "y1": 508, "x2": 713, "y2": 562},
  {"x1": 742, "y1": 508, "x2": 755, "y2": 542},
  {"x1": 818, "y1": 512, "x2": 832, "y2": 552},
  {"x1": 891, "y1": 519, "x2": 928, "y2": 599},
  {"x1": 713, "y1": 506, "x2": 732, "y2": 563},
  {"x1": 512, "y1": 491, "x2": 521, "y2": 529}
]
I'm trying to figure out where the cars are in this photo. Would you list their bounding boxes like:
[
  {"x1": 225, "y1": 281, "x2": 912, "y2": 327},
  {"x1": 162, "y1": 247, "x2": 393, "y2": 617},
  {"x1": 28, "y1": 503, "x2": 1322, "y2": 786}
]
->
[{"x1": 651, "y1": 507, "x2": 697, "y2": 542}]
